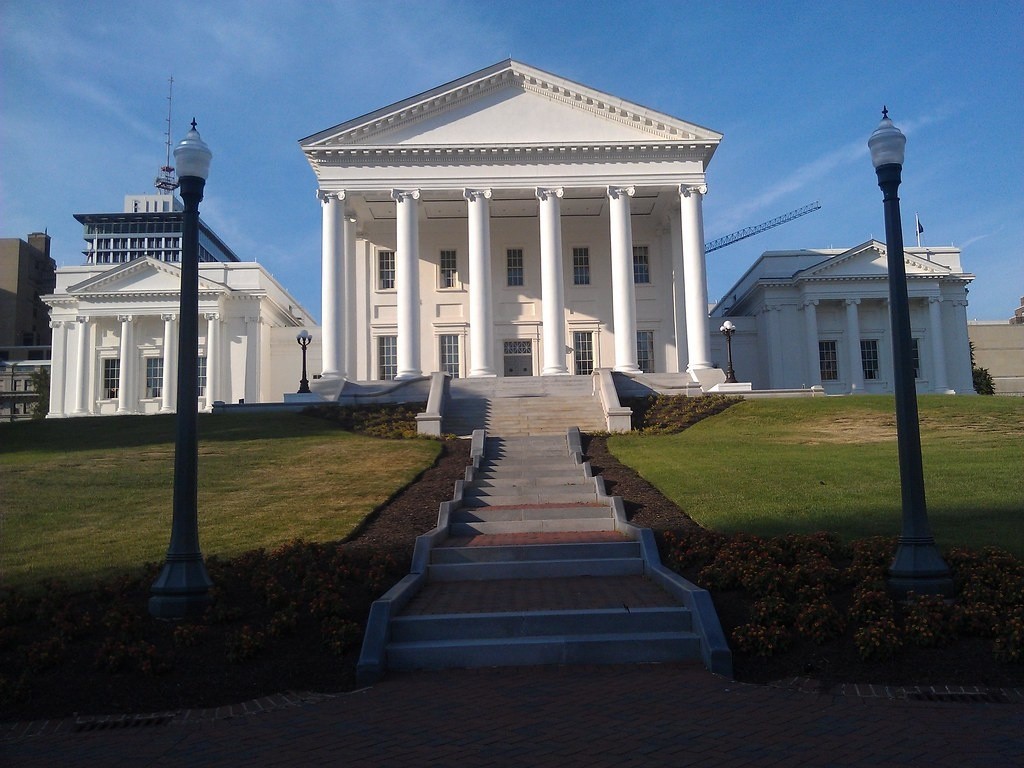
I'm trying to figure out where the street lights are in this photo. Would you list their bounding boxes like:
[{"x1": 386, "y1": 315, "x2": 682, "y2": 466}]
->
[
  {"x1": 142, "y1": 116, "x2": 214, "y2": 617},
  {"x1": 295, "y1": 330, "x2": 313, "y2": 393},
  {"x1": 719, "y1": 319, "x2": 739, "y2": 383},
  {"x1": 861, "y1": 106, "x2": 960, "y2": 600}
]
